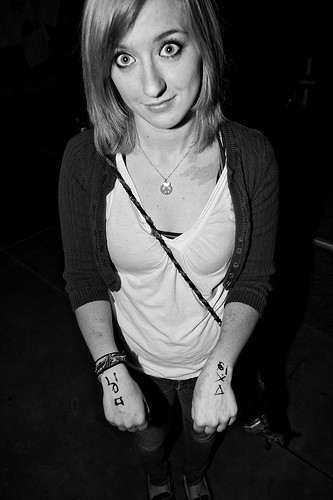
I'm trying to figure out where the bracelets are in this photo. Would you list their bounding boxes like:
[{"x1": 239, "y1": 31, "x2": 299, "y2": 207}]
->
[{"x1": 94, "y1": 351, "x2": 127, "y2": 378}]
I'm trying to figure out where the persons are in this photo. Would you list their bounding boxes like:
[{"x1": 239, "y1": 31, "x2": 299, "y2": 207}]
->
[{"x1": 58, "y1": 0, "x2": 278, "y2": 500}]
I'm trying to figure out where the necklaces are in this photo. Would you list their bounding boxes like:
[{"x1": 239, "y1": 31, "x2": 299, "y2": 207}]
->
[{"x1": 133, "y1": 140, "x2": 201, "y2": 195}]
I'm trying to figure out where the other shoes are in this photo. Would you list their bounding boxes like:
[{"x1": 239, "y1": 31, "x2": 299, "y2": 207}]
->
[
  {"x1": 182, "y1": 475, "x2": 213, "y2": 500},
  {"x1": 143, "y1": 461, "x2": 175, "y2": 500}
]
